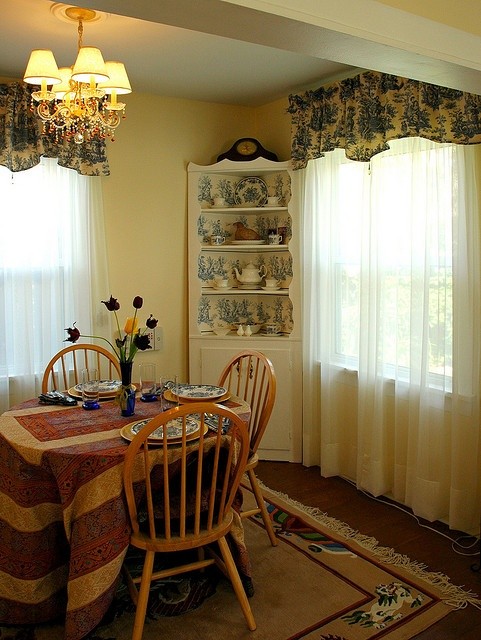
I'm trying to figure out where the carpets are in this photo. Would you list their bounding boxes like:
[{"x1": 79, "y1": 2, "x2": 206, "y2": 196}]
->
[{"x1": 16, "y1": 474, "x2": 481, "y2": 639}]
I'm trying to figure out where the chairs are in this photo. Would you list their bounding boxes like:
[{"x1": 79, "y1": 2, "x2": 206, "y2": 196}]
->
[
  {"x1": 117, "y1": 402, "x2": 257, "y2": 640},
  {"x1": 41, "y1": 344, "x2": 122, "y2": 395},
  {"x1": 218, "y1": 349, "x2": 278, "y2": 547}
]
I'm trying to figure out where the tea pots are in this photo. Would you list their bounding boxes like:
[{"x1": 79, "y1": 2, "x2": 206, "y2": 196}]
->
[{"x1": 232, "y1": 260, "x2": 267, "y2": 283}]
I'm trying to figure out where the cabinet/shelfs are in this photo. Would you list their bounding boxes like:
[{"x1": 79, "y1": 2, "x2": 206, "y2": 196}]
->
[
  {"x1": 186, "y1": 157, "x2": 303, "y2": 340},
  {"x1": 189, "y1": 342, "x2": 304, "y2": 464}
]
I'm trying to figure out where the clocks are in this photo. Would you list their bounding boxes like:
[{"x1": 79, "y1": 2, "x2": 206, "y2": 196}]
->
[{"x1": 217, "y1": 137, "x2": 279, "y2": 162}]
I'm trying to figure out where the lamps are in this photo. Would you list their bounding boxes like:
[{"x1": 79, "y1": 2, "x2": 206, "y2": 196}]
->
[{"x1": 24, "y1": 7, "x2": 133, "y2": 144}]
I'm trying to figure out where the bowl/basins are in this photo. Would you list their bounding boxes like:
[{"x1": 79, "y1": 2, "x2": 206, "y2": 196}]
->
[{"x1": 233, "y1": 322, "x2": 264, "y2": 334}]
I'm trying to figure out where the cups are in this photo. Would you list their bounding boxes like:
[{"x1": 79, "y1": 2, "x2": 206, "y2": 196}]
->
[
  {"x1": 266, "y1": 323, "x2": 282, "y2": 334},
  {"x1": 137, "y1": 362, "x2": 159, "y2": 403},
  {"x1": 213, "y1": 198, "x2": 225, "y2": 205},
  {"x1": 268, "y1": 234, "x2": 282, "y2": 245},
  {"x1": 264, "y1": 278, "x2": 281, "y2": 287},
  {"x1": 214, "y1": 279, "x2": 228, "y2": 288},
  {"x1": 158, "y1": 374, "x2": 179, "y2": 412},
  {"x1": 210, "y1": 234, "x2": 225, "y2": 246},
  {"x1": 266, "y1": 195, "x2": 282, "y2": 205},
  {"x1": 81, "y1": 368, "x2": 100, "y2": 410}
]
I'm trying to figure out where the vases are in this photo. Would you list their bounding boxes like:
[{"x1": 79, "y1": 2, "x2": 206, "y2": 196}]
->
[
  {"x1": 119, "y1": 363, "x2": 136, "y2": 416},
  {"x1": 62, "y1": 294, "x2": 159, "y2": 363}
]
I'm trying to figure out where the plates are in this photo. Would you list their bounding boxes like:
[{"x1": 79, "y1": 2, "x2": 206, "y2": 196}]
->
[
  {"x1": 233, "y1": 176, "x2": 268, "y2": 207},
  {"x1": 261, "y1": 204, "x2": 283, "y2": 207},
  {"x1": 263, "y1": 333, "x2": 283, "y2": 337},
  {"x1": 67, "y1": 381, "x2": 137, "y2": 399},
  {"x1": 130, "y1": 417, "x2": 199, "y2": 440},
  {"x1": 120, "y1": 419, "x2": 209, "y2": 445},
  {"x1": 209, "y1": 206, "x2": 229, "y2": 208},
  {"x1": 212, "y1": 286, "x2": 232, "y2": 290},
  {"x1": 170, "y1": 384, "x2": 227, "y2": 401},
  {"x1": 229, "y1": 239, "x2": 266, "y2": 245},
  {"x1": 73, "y1": 378, "x2": 121, "y2": 397},
  {"x1": 163, "y1": 388, "x2": 232, "y2": 405},
  {"x1": 260, "y1": 287, "x2": 281, "y2": 290}
]
